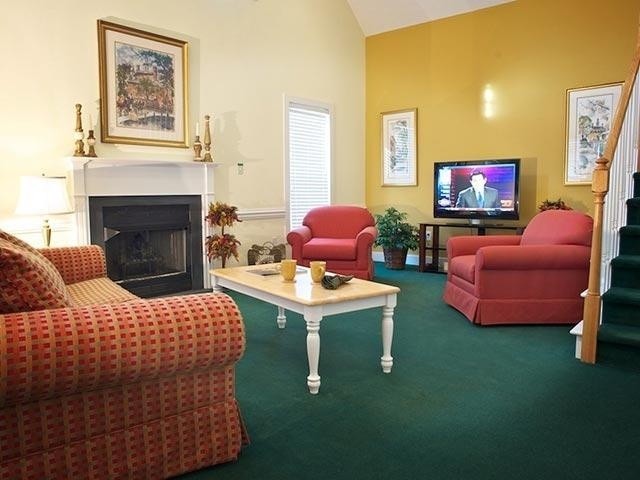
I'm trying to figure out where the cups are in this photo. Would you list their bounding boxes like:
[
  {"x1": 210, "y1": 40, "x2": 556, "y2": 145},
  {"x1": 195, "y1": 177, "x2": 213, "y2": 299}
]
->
[
  {"x1": 275, "y1": 259, "x2": 297, "y2": 281},
  {"x1": 310, "y1": 261, "x2": 327, "y2": 283}
]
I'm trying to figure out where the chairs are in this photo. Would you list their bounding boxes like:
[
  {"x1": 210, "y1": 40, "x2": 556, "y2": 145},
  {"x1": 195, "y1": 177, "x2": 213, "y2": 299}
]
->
[
  {"x1": 445, "y1": 209, "x2": 594, "y2": 326},
  {"x1": 287, "y1": 206, "x2": 377, "y2": 282}
]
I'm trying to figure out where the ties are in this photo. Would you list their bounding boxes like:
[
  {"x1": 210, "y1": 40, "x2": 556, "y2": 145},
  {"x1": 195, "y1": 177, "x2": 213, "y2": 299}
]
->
[{"x1": 477, "y1": 192, "x2": 484, "y2": 207}]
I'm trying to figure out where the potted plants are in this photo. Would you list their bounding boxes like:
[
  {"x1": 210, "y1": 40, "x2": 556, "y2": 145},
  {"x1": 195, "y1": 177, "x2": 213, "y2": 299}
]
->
[{"x1": 374, "y1": 207, "x2": 420, "y2": 269}]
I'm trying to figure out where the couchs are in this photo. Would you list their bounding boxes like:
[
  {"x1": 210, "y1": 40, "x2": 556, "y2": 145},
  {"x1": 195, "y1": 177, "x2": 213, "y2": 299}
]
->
[{"x1": 0, "y1": 244, "x2": 247, "y2": 480}]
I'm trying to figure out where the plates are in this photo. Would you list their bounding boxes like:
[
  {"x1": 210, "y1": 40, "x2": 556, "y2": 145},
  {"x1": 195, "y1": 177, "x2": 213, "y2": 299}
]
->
[
  {"x1": 281, "y1": 280, "x2": 296, "y2": 284},
  {"x1": 311, "y1": 282, "x2": 323, "y2": 286}
]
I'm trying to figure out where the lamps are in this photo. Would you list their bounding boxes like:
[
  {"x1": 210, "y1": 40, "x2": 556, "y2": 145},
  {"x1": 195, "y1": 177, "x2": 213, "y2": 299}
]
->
[{"x1": 13, "y1": 176, "x2": 73, "y2": 246}]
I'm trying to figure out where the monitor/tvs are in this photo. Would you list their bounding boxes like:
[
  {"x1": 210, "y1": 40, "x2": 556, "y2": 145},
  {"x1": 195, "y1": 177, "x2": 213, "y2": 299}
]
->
[{"x1": 433, "y1": 158, "x2": 521, "y2": 221}]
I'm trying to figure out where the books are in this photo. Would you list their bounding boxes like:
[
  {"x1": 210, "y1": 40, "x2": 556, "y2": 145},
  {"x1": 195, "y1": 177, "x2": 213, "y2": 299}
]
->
[{"x1": 246, "y1": 264, "x2": 308, "y2": 277}]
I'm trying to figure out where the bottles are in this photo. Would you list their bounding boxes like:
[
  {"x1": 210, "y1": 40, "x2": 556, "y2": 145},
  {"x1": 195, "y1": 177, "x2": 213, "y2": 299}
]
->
[{"x1": 238, "y1": 160, "x2": 246, "y2": 175}]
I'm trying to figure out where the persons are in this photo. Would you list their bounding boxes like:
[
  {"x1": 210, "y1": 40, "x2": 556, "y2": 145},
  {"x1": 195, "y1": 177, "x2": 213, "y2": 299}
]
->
[{"x1": 455, "y1": 171, "x2": 499, "y2": 209}]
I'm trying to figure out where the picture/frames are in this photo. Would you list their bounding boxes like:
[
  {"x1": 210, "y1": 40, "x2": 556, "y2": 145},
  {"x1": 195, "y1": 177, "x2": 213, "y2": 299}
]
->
[
  {"x1": 564, "y1": 81, "x2": 624, "y2": 183},
  {"x1": 96, "y1": 19, "x2": 189, "y2": 147},
  {"x1": 380, "y1": 108, "x2": 416, "y2": 187}
]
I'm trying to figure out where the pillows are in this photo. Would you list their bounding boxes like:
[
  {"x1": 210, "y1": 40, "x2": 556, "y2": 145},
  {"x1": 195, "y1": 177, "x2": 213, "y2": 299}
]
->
[{"x1": 0, "y1": 230, "x2": 72, "y2": 310}]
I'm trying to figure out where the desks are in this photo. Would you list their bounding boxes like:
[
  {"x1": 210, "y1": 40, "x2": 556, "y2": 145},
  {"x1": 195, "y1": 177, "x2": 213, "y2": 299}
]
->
[{"x1": 418, "y1": 220, "x2": 526, "y2": 273}]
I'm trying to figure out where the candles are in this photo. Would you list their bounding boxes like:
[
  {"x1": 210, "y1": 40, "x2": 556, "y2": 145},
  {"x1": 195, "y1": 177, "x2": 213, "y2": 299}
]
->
[
  {"x1": 195, "y1": 121, "x2": 200, "y2": 137},
  {"x1": 88, "y1": 112, "x2": 94, "y2": 131}
]
